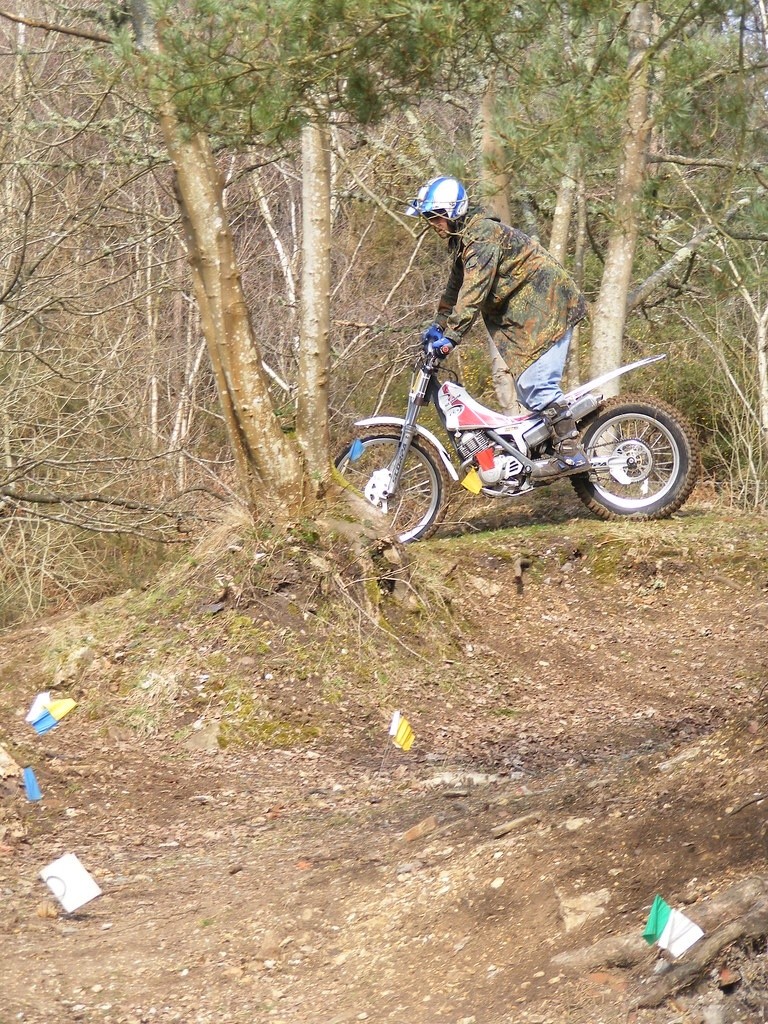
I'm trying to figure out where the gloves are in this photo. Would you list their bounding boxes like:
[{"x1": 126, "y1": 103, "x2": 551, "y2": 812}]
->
[
  {"x1": 422, "y1": 324, "x2": 445, "y2": 345},
  {"x1": 429, "y1": 337, "x2": 455, "y2": 360}
]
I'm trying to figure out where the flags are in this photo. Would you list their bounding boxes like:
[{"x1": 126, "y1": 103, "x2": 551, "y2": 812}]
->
[
  {"x1": 476, "y1": 448, "x2": 495, "y2": 472},
  {"x1": 25, "y1": 691, "x2": 77, "y2": 735},
  {"x1": 462, "y1": 468, "x2": 482, "y2": 494},
  {"x1": 40, "y1": 854, "x2": 102, "y2": 914},
  {"x1": 390, "y1": 711, "x2": 415, "y2": 751},
  {"x1": 639, "y1": 478, "x2": 648, "y2": 495},
  {"x1": 642, "y1": 895, "x2": 704, "y2": 957},
  {"x1": 24, "y1": 768, "x2": 41, "y2": 800},
  {"x1": 347, "y1": 438, "x2": 365, "y2": 462}
]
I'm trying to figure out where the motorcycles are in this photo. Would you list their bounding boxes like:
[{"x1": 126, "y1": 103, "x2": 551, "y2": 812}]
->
[{"x1": 329, "y1": 338, "x2": 702, "y2": 543}]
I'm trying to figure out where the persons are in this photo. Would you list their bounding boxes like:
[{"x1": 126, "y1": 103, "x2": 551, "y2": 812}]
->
[{"x1": 405, "y1": 176, "x2": 592, "y2": 482}]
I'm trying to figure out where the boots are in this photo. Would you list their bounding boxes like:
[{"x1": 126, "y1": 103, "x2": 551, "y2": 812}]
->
[{"x1": 531, "y1": 403, "x2": 590, "y2": 480}]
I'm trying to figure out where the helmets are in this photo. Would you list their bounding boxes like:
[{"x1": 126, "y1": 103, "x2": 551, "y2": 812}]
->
[{"x1": 406, "y1": 176, "x2": 468, "y2": 221}]
